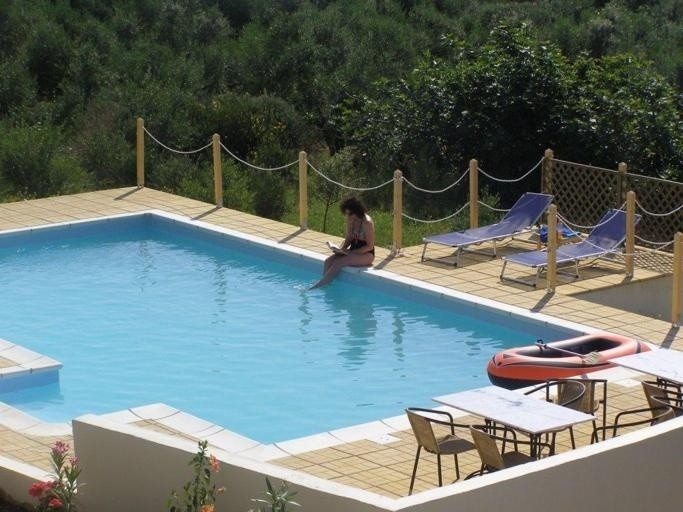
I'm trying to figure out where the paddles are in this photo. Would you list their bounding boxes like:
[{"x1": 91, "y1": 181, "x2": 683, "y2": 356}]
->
[{"x1": 534, "y1": 340, "x2": 600, "y2": 365}]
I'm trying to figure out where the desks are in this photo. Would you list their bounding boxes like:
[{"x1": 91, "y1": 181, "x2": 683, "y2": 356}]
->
[{"x1": 608, "y1": 346, "x2": 682, "y2": 385}]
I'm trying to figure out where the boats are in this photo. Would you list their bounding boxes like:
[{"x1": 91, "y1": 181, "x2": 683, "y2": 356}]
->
[{"x1": 488, "y1": 333, "x2": 654, "y2": 391}]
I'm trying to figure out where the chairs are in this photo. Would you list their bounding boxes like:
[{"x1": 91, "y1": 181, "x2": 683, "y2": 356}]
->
[
  {"x1": 422, "y1": 191, "x2": 642, "y2": 287},
  {"x1": 408, "y1": 378, "x2": 682, "y2": 494}
]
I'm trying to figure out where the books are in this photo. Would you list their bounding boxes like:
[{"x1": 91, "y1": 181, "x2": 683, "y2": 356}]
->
[{"x1": 326, "y1": 241, "x2": 347, "y2": 256}]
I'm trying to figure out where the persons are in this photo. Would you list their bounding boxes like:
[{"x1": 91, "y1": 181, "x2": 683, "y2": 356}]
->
[{"x1": 306, "y1": 196, "x2": 376, "y2": 289}]
